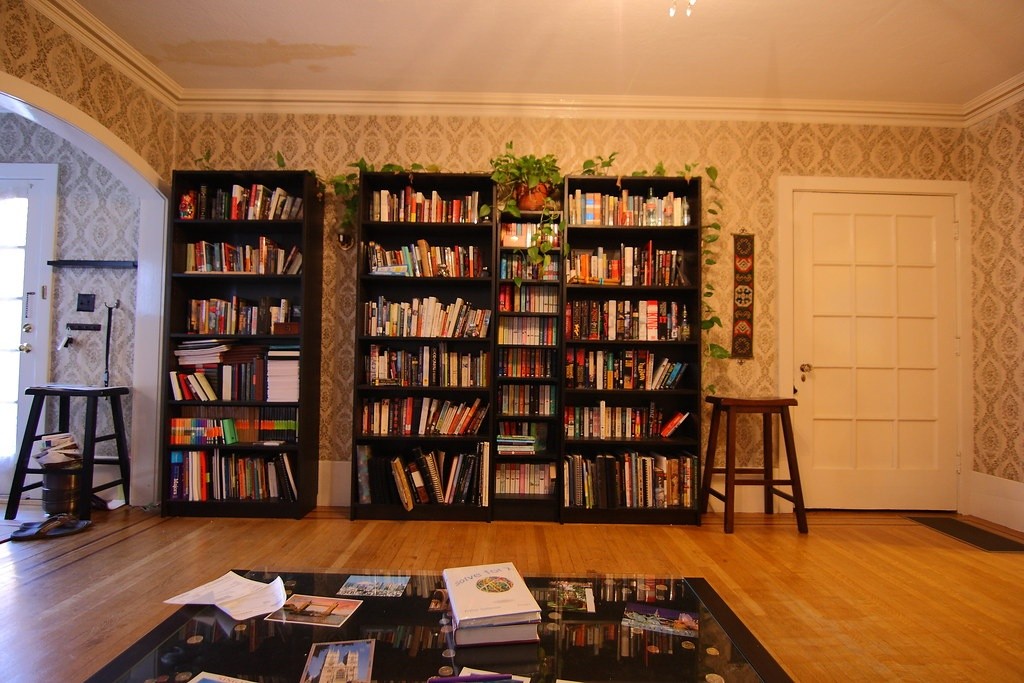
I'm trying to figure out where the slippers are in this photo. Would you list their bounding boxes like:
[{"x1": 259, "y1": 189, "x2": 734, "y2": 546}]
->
[
  {"x1": 10, "y1": 516, "x2": 88, "y2": 541},
  {"x1": 20, "y1": 513, "x2": 92, "y2": 531}
]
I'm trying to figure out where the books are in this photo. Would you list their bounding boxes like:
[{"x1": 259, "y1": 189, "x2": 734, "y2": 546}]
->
[
  {"x1": 181, "y1": 576, "x2": 677, "y2": 667},
  {"x1": 443, "y1": 561, "x2": 541, "y2": 646},
  {"x1": 357, "y1": 185, "x2": 698, "y2": 509},
  {"x1": 169, "y1": 184, "x2": 303, "y2": 500}
]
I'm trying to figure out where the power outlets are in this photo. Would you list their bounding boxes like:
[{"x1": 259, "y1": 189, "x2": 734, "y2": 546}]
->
[{"x1": 76, "y1": 294, "x2": 95, "y2": 311}]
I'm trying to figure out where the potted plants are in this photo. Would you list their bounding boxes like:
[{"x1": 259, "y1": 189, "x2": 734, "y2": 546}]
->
[{"x1": 274, "y1": 140, "x2": 731, "y2": 397}]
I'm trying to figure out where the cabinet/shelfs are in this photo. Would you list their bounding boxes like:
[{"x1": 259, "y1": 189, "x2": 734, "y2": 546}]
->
[
  {"x1": 560, "y1": 175, "x2": 702, "y2": 526},
  {"x1": 159, "y1": 169, "x2": 324, "y2": 520},
  {"x1": 349, "y1": 170, "x2": 497, "y2": 525},
  {"x1": 496, "y1": 211, "x2": 562, "y2": 524}
]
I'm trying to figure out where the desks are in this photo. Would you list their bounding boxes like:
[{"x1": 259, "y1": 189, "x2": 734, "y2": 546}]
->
[
  {"x1": 701, "y1": 395, "x2": 809, "y2": 533},
  {"x1": 4, "y1": 385, "x2": 131, "y2": 520},
  {"x1": 85, "y1": 569, "x2": 795, "y2": 683}
]
